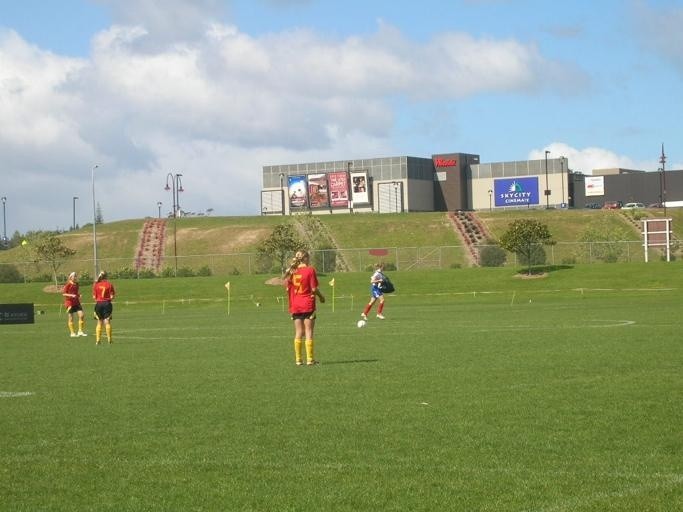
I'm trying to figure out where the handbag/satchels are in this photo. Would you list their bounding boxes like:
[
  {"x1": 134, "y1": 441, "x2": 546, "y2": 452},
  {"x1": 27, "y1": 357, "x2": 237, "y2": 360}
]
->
[{"x1": 381, "y1": 280, "x2": 395, "y2": 292}]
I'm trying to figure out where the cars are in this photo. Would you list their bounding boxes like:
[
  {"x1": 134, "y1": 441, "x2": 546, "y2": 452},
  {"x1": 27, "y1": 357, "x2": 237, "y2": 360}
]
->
[
  {"x1": 621, "y1": 202, "x2": 645, "y2": 209},
  {"x1": 584, "y1": 203, "x2": 601, "y2": 209},
  {"x1": 649, "y1": 203, "x2": 663, "y2": 208},
  {"x1": 602, "y1": 200, "x2": 622, "y2": 209}
]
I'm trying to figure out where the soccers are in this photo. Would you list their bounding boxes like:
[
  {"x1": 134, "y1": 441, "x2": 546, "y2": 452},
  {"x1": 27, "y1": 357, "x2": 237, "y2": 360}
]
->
[{"x1": 358, "y1": 320, "x2": 367, "y2": 328}]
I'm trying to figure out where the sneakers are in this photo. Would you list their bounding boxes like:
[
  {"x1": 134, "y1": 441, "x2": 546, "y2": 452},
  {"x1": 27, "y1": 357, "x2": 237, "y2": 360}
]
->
[
  {"x1": 296, "y1": 362, "x2": 304, "y2": 365},
  {"x1": 376, "y1": 314, "x2": 385, "y2": 320},
  {"x1": 307, "y1": 360, "x2": 317, "y2": 366},
  {"x1": 361, "y1": 312, "x2": 367, "y2": 320},
  {"x1": 71, "y1": 333, "x2": 79, "y2": 338},
  {"x1": 79, "y1": 333, "x2": 88, "y2": 336}
]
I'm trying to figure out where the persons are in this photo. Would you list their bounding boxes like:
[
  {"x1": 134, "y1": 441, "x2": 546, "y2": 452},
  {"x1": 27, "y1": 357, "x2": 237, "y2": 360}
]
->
[
  {"x1": 361, "y1": 264, "x2": 385, "y2": 320},
  {"x1": 62, "y1": 272, "x2": 88, "y2": 337},
  {"x1": 285, "y1": 250, "x2": 325, "y2": 365},
  {"x1": 93, "y1": 270, "x2": 115, "y2": 345}
]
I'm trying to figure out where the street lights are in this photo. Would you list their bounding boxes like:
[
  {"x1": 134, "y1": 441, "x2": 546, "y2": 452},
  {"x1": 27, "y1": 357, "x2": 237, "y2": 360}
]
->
[
  {"x1": 348, "y1": 162, "x2": 353, "y2": 214},
  {"x1": 657, "y1": 167, "x2": 663, "y2": 206},
  {"x1": 73, "y1": 197, "x2": 79, "y2": 231},
  {"x1": 165, "y1": 172, "x2": 184, "y2": 279},
  {"x1": 279, "y1": 173, "x2": 285, "y2": 216},
  {"x1": 559, "y1": 156, "x2": 565, "y2": 208},
  {"x1": 92, "y1": 164, "x2": 98, "y2": 282},
  {"x1": 394, "y1": 182, "x2": 398, "y2": 214},
  {"x1": 545, "y1": 150, "x2": 550, "y2": 210},
  {"x1": 157, "y1": 202, "x2": 161, "y2": 218},
  {"x1": 488, "y1": 189, "x2": 493, "y2": 212},
  {"x1": 1, "y1": 197, "x2": 7, "y2": 248}
]
